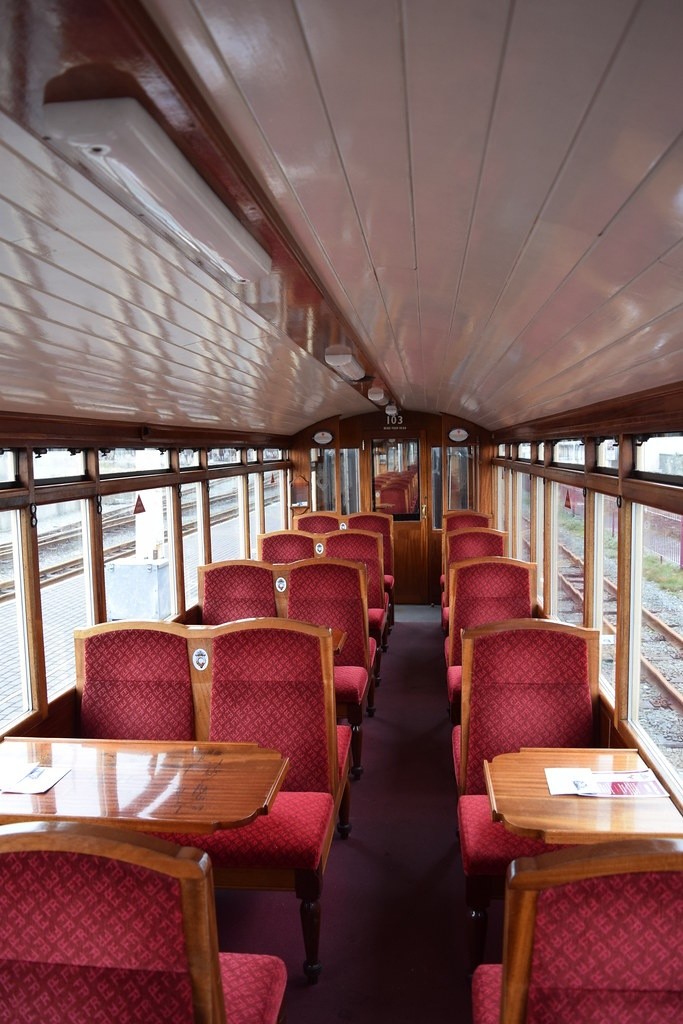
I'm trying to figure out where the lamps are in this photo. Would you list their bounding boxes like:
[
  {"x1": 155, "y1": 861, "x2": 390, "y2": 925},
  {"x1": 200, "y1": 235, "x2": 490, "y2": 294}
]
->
[
  {"x1": 35, "y1": 65, "x2": 275, "y2": 290},
  {"x1": 325, "y1": 353, "x2": 368, "y2": 383},
  {"x1": 385, "y1": 405, "x2": 398, "y2": 418},
  {"x1": 366, "y1": 388, "x2": 390, "y2": 406}
]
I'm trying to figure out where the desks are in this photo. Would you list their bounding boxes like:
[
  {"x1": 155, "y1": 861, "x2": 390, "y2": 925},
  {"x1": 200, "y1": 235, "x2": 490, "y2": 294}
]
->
[
  {"x1": 375, "y1": 504, "x2": 395, "y2": 509},
  {"x1": 0, "y1": 737, "x2": 290, "y2": 833},
  {"x1": 486, "y1": 746, "x2": 683, "y2": 843},
  {"x1": 332, "y1": 628, "x2": 348, "y2": 655}
]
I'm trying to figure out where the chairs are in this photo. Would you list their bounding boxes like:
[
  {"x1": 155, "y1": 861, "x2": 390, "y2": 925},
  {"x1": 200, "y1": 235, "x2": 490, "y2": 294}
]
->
[
  {"x1": 440, "y1": 509, "x2": 683, "y2": 1024},
  {"x1": 0, "y1": 510, "x2": 398, "y2": 1024},
  {"x1": 374, "y1": 464, "x2": 419, "y2": 513}
]
